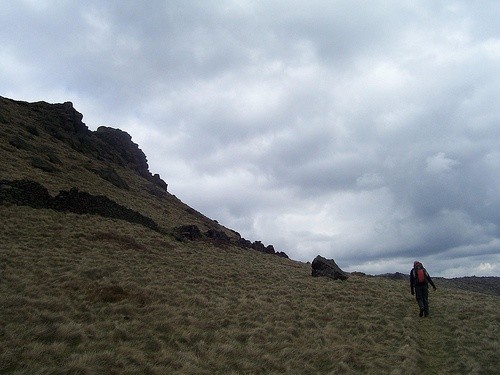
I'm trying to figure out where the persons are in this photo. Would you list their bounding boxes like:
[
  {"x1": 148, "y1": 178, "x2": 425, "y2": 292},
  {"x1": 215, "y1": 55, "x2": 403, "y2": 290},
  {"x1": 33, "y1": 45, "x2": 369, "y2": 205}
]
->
[{"x1": 409, "y1": 261, "x2": 437, "y2": 318}]
